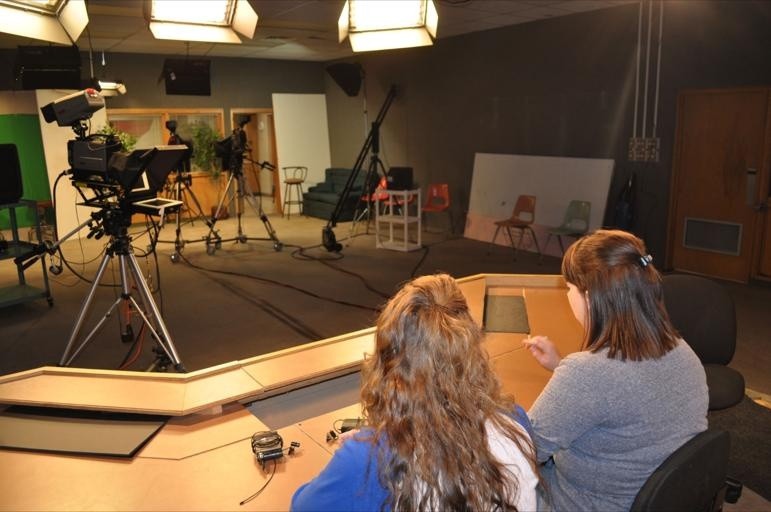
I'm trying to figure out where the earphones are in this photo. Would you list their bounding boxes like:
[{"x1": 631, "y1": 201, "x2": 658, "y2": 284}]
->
[{"x1": 288, "y1": 447, "x2": 295, "y2": 456}]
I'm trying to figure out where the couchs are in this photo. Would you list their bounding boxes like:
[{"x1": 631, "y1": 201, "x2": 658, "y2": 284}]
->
[{"x1": 303, "y1": 163, "x2": 374, "y2": 223}]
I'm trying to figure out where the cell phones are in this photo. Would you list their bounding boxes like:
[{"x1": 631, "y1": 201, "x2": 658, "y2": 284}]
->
[{"x1": 253, "y1": 431, "x2": 284, "y2": 462}]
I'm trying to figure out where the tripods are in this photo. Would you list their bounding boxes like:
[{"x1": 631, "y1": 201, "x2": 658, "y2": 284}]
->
[
  {"x1": 146, "y1": 164, "x2": 222, "y2": 263},
  {"x1": 205, "y1": 169, "x2": 283, "y2": 256},
  {"x1": 59, "y1": 237, "x2": 186, "y2": 372},
  {"x1": 352, "y1": 130, "x2": 403, "y2": 234}
]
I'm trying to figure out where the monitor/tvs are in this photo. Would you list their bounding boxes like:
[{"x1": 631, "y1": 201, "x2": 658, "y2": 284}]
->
[
  {"x1": 387, "y1": 167, "x2": 413, "y2": 189},
  {"x1": 0, "y1": 144, "x2": 23, "y2": 204}
]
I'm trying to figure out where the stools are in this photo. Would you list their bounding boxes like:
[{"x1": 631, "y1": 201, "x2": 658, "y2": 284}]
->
[{"x1": 26, "y1": 200, "x2": 56, "y2": 243}]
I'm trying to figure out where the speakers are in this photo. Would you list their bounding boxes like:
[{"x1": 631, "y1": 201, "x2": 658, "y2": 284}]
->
[
  {"x1": 165, "y1": 59, "x2": 210, "y2": 95},
  {"x1": 326, "y1": 63, "x2": 361, "y2": 96},
  {"x1": 18, "y1": 45, "x2": 80, "y2": 90}
]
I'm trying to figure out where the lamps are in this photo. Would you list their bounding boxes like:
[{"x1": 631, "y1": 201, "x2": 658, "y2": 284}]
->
[
  {"x1": 142, "y1": 0, "x2": 260, "y2": 46},
  {"x1": 335, "y1": 0, "x2": 439, "y2": 52},
  {"x1": 0, "y1": 1, "x2": 93, "y2": 46}
]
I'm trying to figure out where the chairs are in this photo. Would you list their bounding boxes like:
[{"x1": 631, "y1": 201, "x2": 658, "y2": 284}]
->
[
  {"x1": 385, "y1": 194, "x2": 416, "y2": 217},
  {"x1": 280, "y1": 166, "x2": 309, "y2": 220},
  {"x1": 650, "y1": 264, "x2": 768, "y2": 507},
  {"x1": 420, "y1": 182, "x2": 456, "y2": 238},
  {"x1": 625, "y1": 424, "x2": 732, "y2": 512},
  {"x1": 359, "y1": 174, "x2": 389, "y2": 221},
  {"x1": 540, "y1": 199, "x2": 591, "y2": 264},
  {"x1": 488, "y1": 195, "x2": 541, "y2": 262}
]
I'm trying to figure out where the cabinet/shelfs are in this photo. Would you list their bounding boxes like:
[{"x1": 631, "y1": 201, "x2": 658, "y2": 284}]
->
[{"x1": 0, "y1": 198, "x2": 57, "y2": 315}]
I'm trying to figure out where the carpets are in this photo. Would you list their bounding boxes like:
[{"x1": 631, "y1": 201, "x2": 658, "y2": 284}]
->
[{"x1": 709, "y1": 393, "x2": 770, "y2": 502}]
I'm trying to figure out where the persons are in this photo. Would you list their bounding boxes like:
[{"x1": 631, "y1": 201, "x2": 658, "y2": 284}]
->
[
  {"x1": 523, "y1": 230, "x2": 710, "y2": 512},
  {"x1": 290, "y1": 273, "x2": 532, "y2": 512}
]
